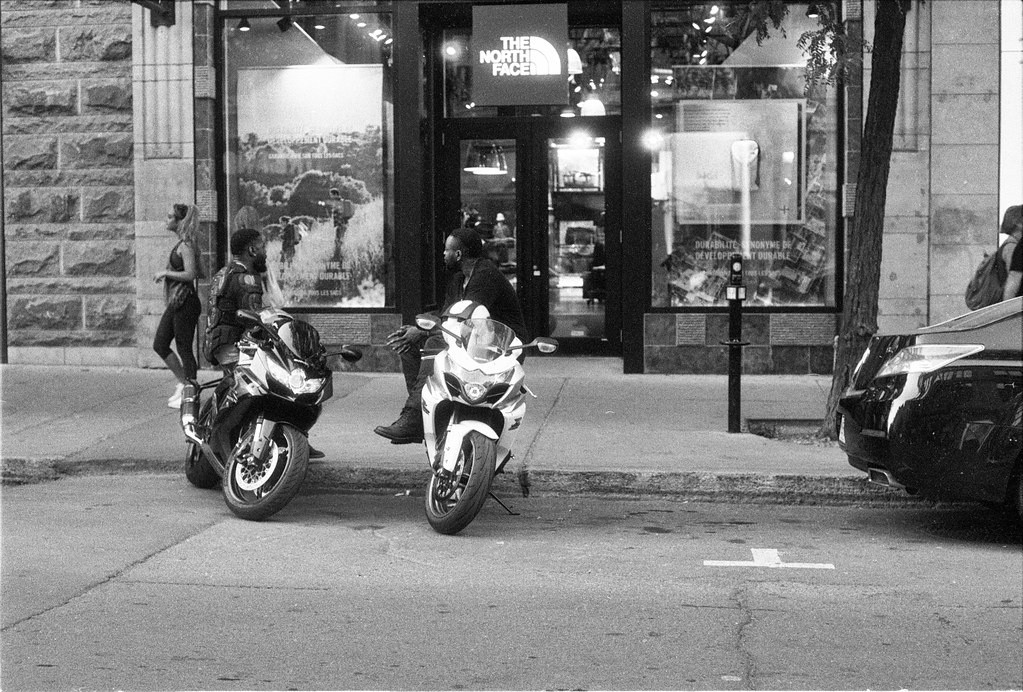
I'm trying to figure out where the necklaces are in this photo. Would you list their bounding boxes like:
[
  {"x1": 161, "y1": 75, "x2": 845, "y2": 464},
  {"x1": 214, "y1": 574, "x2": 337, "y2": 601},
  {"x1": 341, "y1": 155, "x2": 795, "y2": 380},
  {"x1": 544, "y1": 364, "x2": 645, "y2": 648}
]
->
[{"x1": 1011, "y1": 234, "x2": 1019, "y2": 242}]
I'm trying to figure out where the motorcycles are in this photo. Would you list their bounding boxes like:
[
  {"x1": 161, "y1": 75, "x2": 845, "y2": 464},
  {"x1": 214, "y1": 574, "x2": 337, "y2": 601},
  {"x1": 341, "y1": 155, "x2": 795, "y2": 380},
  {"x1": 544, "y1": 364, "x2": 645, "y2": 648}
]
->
[
  {"x1": 415, "y1": 313, "x2": 560, "y2": 537},
  {"x1": 179, "y1": 309, "x2": 364, "y2": 521}
]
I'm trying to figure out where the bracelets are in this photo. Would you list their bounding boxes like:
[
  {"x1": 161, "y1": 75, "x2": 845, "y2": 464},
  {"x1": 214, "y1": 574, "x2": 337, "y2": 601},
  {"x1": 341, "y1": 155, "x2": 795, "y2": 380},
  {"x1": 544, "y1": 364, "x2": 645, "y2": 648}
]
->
[{"x1": 427, "y1": 331, "x2": 429, "y2": 338}]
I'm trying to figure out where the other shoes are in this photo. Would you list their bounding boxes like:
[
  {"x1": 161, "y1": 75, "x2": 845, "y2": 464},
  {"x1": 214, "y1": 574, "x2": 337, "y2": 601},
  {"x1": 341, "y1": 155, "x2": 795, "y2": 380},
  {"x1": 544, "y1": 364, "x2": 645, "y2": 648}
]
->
[
  {"x1": 307, "y1": 444, "x2": 326, "y2": 458},
  {"x1": 168, "y1": 383, "x2": 184, "y2": 409}
]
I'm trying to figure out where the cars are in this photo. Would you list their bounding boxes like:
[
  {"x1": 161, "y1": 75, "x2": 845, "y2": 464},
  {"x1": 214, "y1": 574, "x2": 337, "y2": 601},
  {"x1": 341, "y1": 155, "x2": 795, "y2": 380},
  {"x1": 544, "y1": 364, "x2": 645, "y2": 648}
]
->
[{"x1": 838, "y1": 294, "x2": 1023, "y2": 530}]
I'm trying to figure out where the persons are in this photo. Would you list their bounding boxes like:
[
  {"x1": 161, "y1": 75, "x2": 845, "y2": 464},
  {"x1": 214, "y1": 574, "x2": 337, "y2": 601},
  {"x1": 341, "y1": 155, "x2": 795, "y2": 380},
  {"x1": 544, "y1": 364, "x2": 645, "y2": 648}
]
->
[
  {"x1": 374, "y1": 228, "x2": 527, "y2": 444},
  {"x1": 996, "y1": 204, "x2": 1023, "y2": 300},
  {"x1": 278, "y1": 216, "x2": 295, "y2": 277},
  {"x1": 320, "y1": 189, "x2": 349, "y2": 262},
  {"x1": 152, "y1": 203, "x2": 202, "y2": 408},
  {"x1": 493, "y1": 213, "x2": 512, "y2": 266},
  {"x1": 202, "y1": 229, "x2": 325, "y2": 458}
]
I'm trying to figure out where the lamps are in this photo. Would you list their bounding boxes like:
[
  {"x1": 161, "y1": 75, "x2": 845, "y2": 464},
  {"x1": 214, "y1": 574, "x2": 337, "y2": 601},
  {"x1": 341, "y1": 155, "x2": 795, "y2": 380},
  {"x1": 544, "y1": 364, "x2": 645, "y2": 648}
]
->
[
  {"x1": 462, "y1": 139, "x2": 509, "y2": 175},
  {"x1": 237, "y1": 18, "x2": 251, "y2": 32}
]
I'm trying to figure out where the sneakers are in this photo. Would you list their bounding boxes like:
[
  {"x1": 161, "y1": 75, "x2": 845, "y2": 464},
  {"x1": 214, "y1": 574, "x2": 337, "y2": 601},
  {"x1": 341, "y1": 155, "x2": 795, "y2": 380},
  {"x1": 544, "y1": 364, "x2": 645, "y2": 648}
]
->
[{"x1": 374, "y1": 407, "x2": 426, "y2": 444}]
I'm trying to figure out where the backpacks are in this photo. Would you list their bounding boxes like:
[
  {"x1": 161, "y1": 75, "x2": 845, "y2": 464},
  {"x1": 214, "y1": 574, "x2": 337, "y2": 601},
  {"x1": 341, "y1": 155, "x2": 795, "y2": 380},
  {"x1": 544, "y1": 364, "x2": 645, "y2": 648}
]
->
[
  {"x1": 965, "y1": 240, "x2": 1019, "y2": 309},
  {"x1": 343, "y1": 200, "x2": 355, "y2": 219}
]
